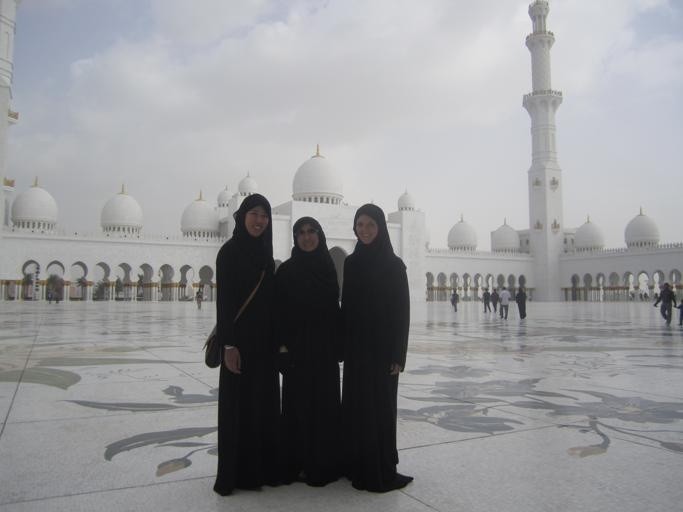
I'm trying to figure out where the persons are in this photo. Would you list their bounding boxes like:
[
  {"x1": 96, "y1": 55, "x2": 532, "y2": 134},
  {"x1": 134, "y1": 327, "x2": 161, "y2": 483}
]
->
[
  {"x1": 450, "y1": 288, "x2": 459, "y2": 314},
  {"x1": 515, "y1": 287, "x2": 527, "y2": 320},
  {"x1": 340, "y1": 201, "x2": 415, "y2": 493},
  {"x1": 480, "y1": 287, "x2": 491, "y2": 315},
  {"x1": 497, "y1": 286, "x2": 511, "y2": 319},
  {"x1": 270, "y1": 216, "x2": 349, "y2": 488},
  {"x1": 652, "y1": 281, "x2": 676, "y2": 328},
  {"x1": 675, "y1": 300, "x2": 682, "y2": 326},
  {"x1": 194, "y1": 287, "x2": 202, "y2": 309},
  {"x1": 214, "y1": 193, "x2": 296, "y2": 498},
  {"x1": 491, "y1": 288, "x2": 499, "y2": 313}
]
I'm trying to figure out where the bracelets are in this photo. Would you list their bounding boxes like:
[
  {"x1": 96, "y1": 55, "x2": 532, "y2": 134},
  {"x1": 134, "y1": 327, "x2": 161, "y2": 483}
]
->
[{"x1": 222, "y1": 345, "x2": 235, "y2": 350}]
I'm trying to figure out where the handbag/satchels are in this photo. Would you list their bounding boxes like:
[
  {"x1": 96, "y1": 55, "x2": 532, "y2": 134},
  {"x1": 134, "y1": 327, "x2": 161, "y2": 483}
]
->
[{"x1": 201, "y1": 323, "x2": 222, "y2": 369}]
